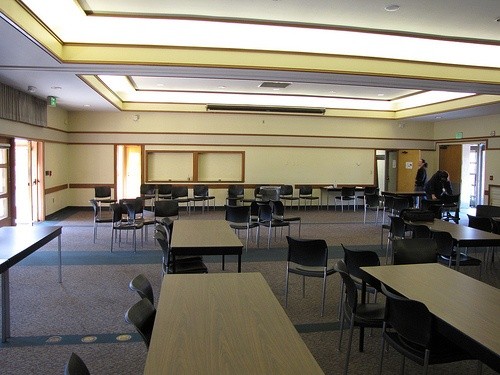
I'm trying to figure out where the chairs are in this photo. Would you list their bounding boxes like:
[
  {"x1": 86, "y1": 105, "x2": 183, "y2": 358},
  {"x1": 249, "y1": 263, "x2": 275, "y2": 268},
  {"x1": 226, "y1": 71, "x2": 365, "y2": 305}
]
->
[{"x1": 64, "y1": 184, "x2": 499, "y2": 375}]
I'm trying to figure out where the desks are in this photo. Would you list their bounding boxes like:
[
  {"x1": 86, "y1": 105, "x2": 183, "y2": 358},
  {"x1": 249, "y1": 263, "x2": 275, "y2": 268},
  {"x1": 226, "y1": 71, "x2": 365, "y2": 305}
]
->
[
  {"x1": 404, "y1": 218, "x2": 500, "y2": 271},
  {"x1": 382, "y1": 191, "x2": 426, "y2": 224},
  {"x1": 0, "y1": 226, "x2": 63, "y2": 338},
  {"x1": 143, "y1": 272, "x2": 325, "y2": 375},
  {"x1": 359, "y1": 263, "x2": 500, "y2": 375},
  {"x1": 171, "y1": 220, "x2": 243, "y2": 274}
]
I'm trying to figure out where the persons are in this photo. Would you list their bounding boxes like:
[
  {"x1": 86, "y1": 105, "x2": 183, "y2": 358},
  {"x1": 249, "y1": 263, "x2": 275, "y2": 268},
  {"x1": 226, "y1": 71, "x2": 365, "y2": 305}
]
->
[
  {"x1": 425, "y1": 170, "x2": 454, "y2": 201},
  {"x1": 414, "y1": 159, "x2": 428, "y2": 210}
]
